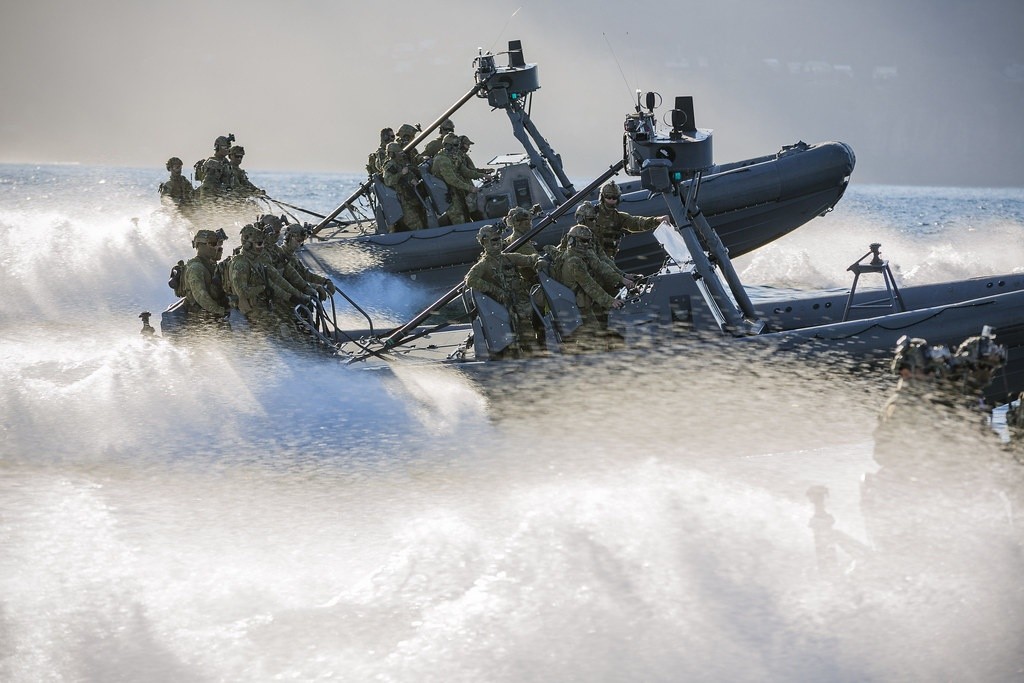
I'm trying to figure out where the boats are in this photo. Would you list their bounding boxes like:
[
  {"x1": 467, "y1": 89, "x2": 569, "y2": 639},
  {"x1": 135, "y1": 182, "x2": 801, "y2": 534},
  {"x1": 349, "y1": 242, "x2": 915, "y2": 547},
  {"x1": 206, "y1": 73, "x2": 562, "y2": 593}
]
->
[
  {"x1": 135, "y1": 160, "x2": 1023, "y2": 395},
  {"x1": 275, "y1": 38, "x2": 858, "y2": 325}
]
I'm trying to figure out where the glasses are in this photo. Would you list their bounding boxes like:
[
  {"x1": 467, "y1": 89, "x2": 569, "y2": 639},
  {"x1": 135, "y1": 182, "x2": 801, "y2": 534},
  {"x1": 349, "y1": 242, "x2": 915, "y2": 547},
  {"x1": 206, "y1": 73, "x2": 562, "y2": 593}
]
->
[
  {"x1": 588, "y1": 218, "x2": 597, "y2": 221},
  {"x1": 491, "y1": 237, "x2": 501, "y2": 241},
  {"x1": 387, "y1": 135, "x2": 395, "y2": 138},
  {"x1": 578, "y1": 237, "x2": 590, "y2": 244},
  {"x1": 603, "y1": 195, "x2": 617, "y2": 200}
]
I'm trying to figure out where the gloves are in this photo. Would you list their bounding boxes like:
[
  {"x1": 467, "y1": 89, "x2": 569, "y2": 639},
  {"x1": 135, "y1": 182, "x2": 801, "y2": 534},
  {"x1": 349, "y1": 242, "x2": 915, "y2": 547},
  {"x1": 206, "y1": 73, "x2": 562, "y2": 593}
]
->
[{"x1": 301, "y1": 279, "x2": 336, "y2": 305}]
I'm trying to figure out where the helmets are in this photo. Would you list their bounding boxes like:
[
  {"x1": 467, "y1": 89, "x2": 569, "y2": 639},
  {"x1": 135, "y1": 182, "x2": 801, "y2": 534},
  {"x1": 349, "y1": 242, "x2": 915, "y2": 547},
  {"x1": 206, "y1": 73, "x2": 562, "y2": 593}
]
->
[
  {"x1": 567, "y1": 224, "x2": 592, "y2": 241},
  {"x1": 165, "y1": 136, "x2": 245, "y2": 174},
  {"x1": 440, "y1": 120, "x2": 454, "y2": 134},
  {"x1": 458, "y1": 136, "x2": 474, "y2": 145},
  {"x1": 193, "y1": 213, "x2": 308, "y2": 249},
  {"x1": 476, "y1": 225, "x2": 498, "y2": 245},
  {"x1": 397, "y1": 124, "x2": 416, "y2": 138},
  {"x1": 574, "y1": 202, "x2": 597, "y2": 220},
  {"x1": 386, "y1": 143, "x2": 403, "y2": 156},
  {"x1": 600, "y1": 184, "x2": 621, "y2": 197},
  {"x1": 508, "y1": 207, "x2": 531, "y2": 227},
  {"x1": 442, "y1": 132, "x2": 460, "y2": 148}
]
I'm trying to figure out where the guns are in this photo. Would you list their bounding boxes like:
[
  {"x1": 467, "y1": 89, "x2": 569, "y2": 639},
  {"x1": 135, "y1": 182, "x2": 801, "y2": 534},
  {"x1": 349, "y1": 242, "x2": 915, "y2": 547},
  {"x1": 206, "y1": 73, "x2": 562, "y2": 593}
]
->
[
  {"x1": 387, "y1": 165, "x2": 420, "y2": 203},
  {"x1": 213, "y1": 276, "x2": 227, "y2": 307},
  {"x1": 262, "y1": 263, "x2": 273, "y2": 309}
]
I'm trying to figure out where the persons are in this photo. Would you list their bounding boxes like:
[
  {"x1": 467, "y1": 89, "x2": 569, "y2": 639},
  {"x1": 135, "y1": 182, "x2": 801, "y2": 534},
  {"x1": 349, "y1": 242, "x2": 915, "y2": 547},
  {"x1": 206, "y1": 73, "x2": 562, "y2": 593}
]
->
[
  {"x1": 1005, "y1": 390, "x2": 1024, "y2": 457},
  {"x1": 464, "y1": 223, "x2": 542, "y2": 361},
  {"x1": 158, "y1": 134, "x2": 339, "y2": 362},
  {"x1": 956, "y1": 325, "x2": 1005, "y2": 398},
  {"x1": 546, "y1": 224, "x2": 629, "y2": 347},
  {"x1": 593, "y1": 182, "x2": 672, "y2": 282},
  {"x1": 502, "y1": 201, "x2": 556, "y2": 261},
  {"x1": 366, "y1": 115, "x2": 493, "y2": 230},
  {"x1": 889, "y1": 335, "x2": 940, "y2": 394},
  {"x1": 564, "y1": 201, "x2": 643, "y2": 305}
]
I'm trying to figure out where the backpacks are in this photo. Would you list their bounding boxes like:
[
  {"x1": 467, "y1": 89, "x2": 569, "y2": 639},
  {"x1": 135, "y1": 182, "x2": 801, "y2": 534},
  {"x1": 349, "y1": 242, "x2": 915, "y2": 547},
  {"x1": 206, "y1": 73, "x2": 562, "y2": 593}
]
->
[
  {"x1": 168, "y1": 258, "x2": 213, "y2": 298},
  {"x1": 366, "y1": 147, "x2": 384, "y2": 173}
]
